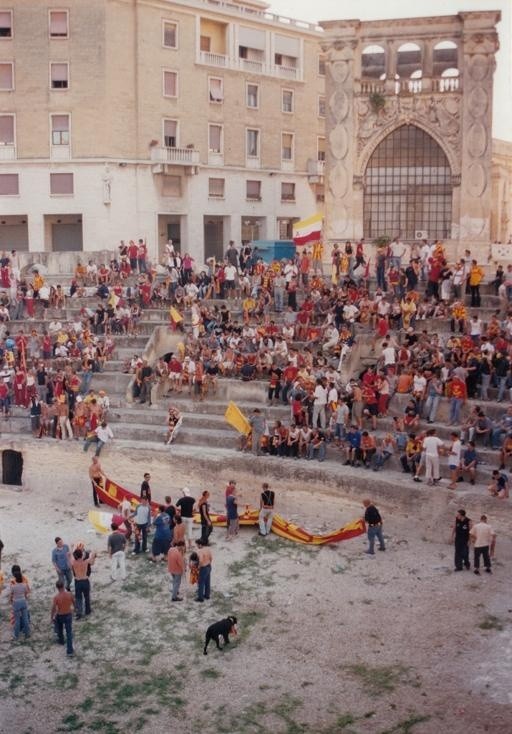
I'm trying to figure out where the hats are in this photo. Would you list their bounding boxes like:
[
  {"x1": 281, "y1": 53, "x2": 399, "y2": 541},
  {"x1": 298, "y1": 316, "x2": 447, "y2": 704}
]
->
[
  {"x1": 183, "y1": 487, "x2": 190, "y2": 497},
  {"x1": 76, "y1": 396, "x2": 82, "y2": 403}
]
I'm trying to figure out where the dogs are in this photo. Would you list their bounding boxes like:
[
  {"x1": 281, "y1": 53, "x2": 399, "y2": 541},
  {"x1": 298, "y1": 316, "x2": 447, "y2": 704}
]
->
[{"x1": 204, "y1": 615, "x2": 238, "y2": 655}]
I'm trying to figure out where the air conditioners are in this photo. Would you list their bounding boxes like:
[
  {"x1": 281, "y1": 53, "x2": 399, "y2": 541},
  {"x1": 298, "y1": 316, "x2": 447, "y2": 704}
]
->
[{"x1": 414, "y1": 231, "x2": 429, "y2": 239}]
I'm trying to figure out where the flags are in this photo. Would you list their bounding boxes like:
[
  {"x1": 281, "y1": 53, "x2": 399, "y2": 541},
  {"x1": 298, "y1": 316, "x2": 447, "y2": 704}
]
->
[{"x1": 292, "y1": 212, "x2": 323, "y2": 245}]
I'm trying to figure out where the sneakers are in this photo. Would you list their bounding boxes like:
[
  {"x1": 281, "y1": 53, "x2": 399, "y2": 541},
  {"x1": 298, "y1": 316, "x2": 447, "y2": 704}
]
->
[
  {"x1": 194, "y1": 597, "x2": 204, "y2": 602},
  {"x1": 172, "y1": 597, "x2": 183, "y2": 601}
]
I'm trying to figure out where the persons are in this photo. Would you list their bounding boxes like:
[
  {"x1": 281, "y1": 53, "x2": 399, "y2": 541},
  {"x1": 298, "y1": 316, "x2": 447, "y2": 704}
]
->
[{"x1": 1, "y1": 240, "x2": 511, "y2": 654}]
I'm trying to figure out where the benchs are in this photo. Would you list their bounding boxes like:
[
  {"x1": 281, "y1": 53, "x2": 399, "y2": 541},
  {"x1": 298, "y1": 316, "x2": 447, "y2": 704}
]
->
[{"x1": 0, "y1": 262, "x2": 510, "y2": 503}]
